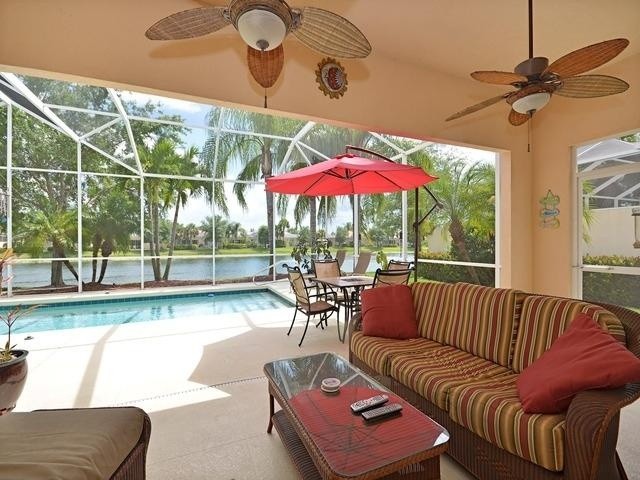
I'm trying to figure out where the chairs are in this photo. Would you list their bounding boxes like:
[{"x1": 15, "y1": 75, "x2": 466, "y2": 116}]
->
[{"x1": 284, "y1": 249, "x2": 414, "y2": 348}]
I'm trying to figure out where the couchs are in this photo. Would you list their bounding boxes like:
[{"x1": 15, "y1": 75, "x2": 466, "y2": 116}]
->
[{"x1": 347, "y1": 281, "x2": 640, "y2": 480}]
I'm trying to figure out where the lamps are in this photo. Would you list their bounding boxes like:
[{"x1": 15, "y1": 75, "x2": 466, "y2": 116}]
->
[
  {"x1": 510, "y1": 90, "x2": 552, "y2": 119},
  {"x1": 235, "y1": 8, "x2": 286, "y2": 52}
]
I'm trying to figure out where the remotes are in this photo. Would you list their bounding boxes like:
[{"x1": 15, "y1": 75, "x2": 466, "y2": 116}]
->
[
  {"x1": 361, "y1": 403, "x2": 403, "y2": 420},
  {"x1": 350, "y1": 394, "x2": 389, "y2": 413}
]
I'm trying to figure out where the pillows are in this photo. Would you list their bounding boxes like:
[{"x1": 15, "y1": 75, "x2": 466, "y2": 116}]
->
[
  {"x1": 515, "y1": 312, "x2": 640, "y2": 414},
  {"x1": 361, "y1": 284, "x2": 420, "y2": 339}
]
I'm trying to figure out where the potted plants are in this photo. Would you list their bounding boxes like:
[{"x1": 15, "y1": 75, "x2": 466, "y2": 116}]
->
[{"x1": 0, "y1": 247, "x2": 52, "y2": 417}]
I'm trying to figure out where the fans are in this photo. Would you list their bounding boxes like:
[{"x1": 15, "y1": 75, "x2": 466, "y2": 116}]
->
[
  {"x1": 145, "y1": 0, "x2": 372, "y2": 87},
  {"x1": 445, "y1": 0, "x2": 630, "y2": 153}
]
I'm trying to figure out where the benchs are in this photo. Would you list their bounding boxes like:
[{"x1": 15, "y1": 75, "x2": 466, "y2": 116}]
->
[{"x1": 0, "y1": 406, "x2": 151, "y2": 480}]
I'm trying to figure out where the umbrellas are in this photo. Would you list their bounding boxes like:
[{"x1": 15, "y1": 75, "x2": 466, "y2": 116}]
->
[{"x1": 264, "y1": 144, "x2": 441, "y2": 282}]
